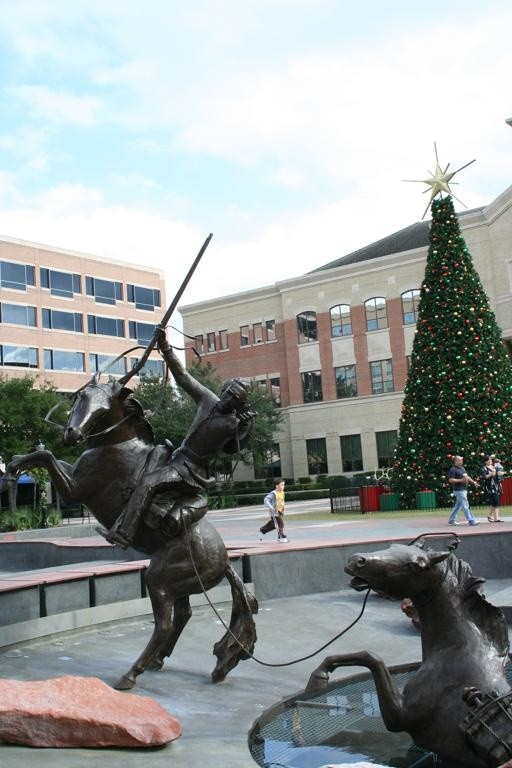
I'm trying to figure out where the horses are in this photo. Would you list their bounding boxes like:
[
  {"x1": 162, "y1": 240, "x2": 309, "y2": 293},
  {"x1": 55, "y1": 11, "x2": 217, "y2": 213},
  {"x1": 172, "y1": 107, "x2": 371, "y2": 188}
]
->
[
  {"x1": 304, "y1": 535, "x2": 512, "y2": 768},
  {"x1": 0, "y1": 371, "x2": 259, "y2": 691}
]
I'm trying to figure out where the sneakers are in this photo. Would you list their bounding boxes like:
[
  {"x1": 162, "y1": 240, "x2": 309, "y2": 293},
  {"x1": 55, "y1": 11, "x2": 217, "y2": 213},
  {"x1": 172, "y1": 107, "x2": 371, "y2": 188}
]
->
[
  {"x1": 254, "y1": 528, "x2": 288, "y2": 545},
  {"x1": 448, "y1": 517, "x2": 505, "y2": 527}
]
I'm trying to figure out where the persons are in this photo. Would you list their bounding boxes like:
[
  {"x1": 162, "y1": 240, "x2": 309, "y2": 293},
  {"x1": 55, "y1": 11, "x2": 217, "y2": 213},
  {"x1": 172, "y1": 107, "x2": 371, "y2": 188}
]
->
[
  {"x1": 492, "y1": 458, "x2": 504, "y2": 483},
  {"x1": 446, "y1": 454, "x2": 481, "y2": 527},
  {"x1": 93, "y1": 324, "x2": 259, "y2": 552},
  {"x1": 478, "y1": 455, "x2": 508, "y2": 523},
  {"x1": 256, "y1": 478, "x2": 289, "y2": 543}
]
